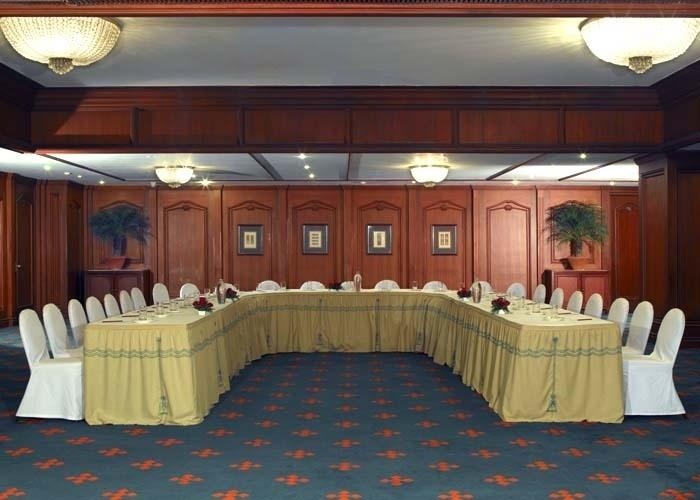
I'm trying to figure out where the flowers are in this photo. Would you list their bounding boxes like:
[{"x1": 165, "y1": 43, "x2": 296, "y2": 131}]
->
[
  {"x1": 489, "y1": 297, "x2": 510, "y2": 315},
  {"x1": 457, "y1": 287, "x2": 471, "y2": 300},
  {"x1": 193, "y1": 296, "x2": 214, "y2": 315},
  {"x1": 226, "y1": 288, "x2": 239, "y2": 302}
]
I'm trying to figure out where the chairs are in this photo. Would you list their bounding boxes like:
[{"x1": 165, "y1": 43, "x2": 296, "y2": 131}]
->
[
  {"x1": 216, "y1": 278, "x2": 448, "y2": 291},
  {"x1": 43, "y1": 302, "x2": 84, "y2": 358},
  {"x1": 621, "y1": 307, "x2": 689, "y2": 420},
  {"x1": 469, "y1": 279, "x2": 604, "y2": 319},
  {"x1": 607, "y1": 296, "x2": 629, "y2": 342},
  {"x1": 14, "y1": 308, "x2": 85, "y2": 420},
  {"x1": 618, "y1": 300, "x2": 654, "y2": 353},
  {"x1": 85, "y1": 282, "x2": 201, "y2": 322}
]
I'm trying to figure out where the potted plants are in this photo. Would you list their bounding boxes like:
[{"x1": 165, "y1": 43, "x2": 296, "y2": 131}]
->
[
  {"x1": 88, "y1": 204, "x2": 156, "y2": 268},
  {"x1": 544, "y1": 197, "x2": 611, "y2": 270}
]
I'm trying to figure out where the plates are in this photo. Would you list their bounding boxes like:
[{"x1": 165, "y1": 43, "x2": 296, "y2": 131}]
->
[
  {"x1": 134, "y1": 319, "x2": 151, "y2": 324},
  {"x1": 545, "y1": 316, "x2": 564, "y2": 322}
]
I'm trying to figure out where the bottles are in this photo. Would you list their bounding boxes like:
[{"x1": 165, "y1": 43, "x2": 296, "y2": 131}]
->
[
  {"x1": 354, "y1": 271, "x2": 361, "y2": 291},
  {"x1": 218, "y1": 279, "x2": 226, "y2": 304},
  {"x1": 472, "y1": 279, "x2": 482, "y2": 302},
  {"x1": 480, "y1": 284, "x2": 558, "y2": 319}
]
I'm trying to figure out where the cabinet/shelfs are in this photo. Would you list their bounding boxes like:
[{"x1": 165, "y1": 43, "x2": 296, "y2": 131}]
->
[
  {"x1": 545, "y1": 269, "x2": 611, "y2": 313},
  {"x1": 83, "y1": 269, "x2": 152, "y2": 309}
]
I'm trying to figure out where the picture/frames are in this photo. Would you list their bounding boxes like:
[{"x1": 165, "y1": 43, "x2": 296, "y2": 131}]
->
[
  {"x1": 366, "y1": 222, "x2": 392, "y2": 255},
  {"x1": 430, "y1": 224, "x2": 457, "y2": 256},
  {"x1": 236, "y1": 222, "x2": 265, "y2": 256},
  {"x1": 302, "y1": 222, "x2": 328, "y2": 255}
]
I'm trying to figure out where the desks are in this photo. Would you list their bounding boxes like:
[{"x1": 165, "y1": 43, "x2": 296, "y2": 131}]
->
[
  {"x1": 245, "y1": 290, "x2": 443, "y2": 366},
  {"x1": 84, "y1": 290, "x2": 246, "y2": 431},
  {"x1": 443, "y1": 287, "x2": 627, "y2": 426}
]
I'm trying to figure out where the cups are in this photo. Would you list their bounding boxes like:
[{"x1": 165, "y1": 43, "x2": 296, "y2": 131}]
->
[
  {"x1": 412, "y1": 280, "x2": 418, "y2": 290},
  {"x1": 234, "y1": 280, "x2": 239, "y2": 291},
  {"x1": 138, "y1": 288, "x2": 216, "y2": 321},
  {"x1": 307, "y1": 279, "x2": 312, "y2": 290},
  {"x1": 281, "y1": 281, "x2": 286, "y2": 290},
  {"x1": 438, "y1": 280, "x2": 444, "y2": 291}
]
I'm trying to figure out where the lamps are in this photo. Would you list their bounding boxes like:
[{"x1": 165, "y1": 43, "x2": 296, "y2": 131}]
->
[
  {"x1": 578, "y1": 15, "x2": 699, "y2": 78},
  {"x1": 0, "y1": 14, "x2": 123, "y2": 76},
  {"x1": 407, "y1": 164, "x2": 448, "y2": 189},
  {"x1": 153, "y1": 166, "x2": 195, "y2": 191}
]
